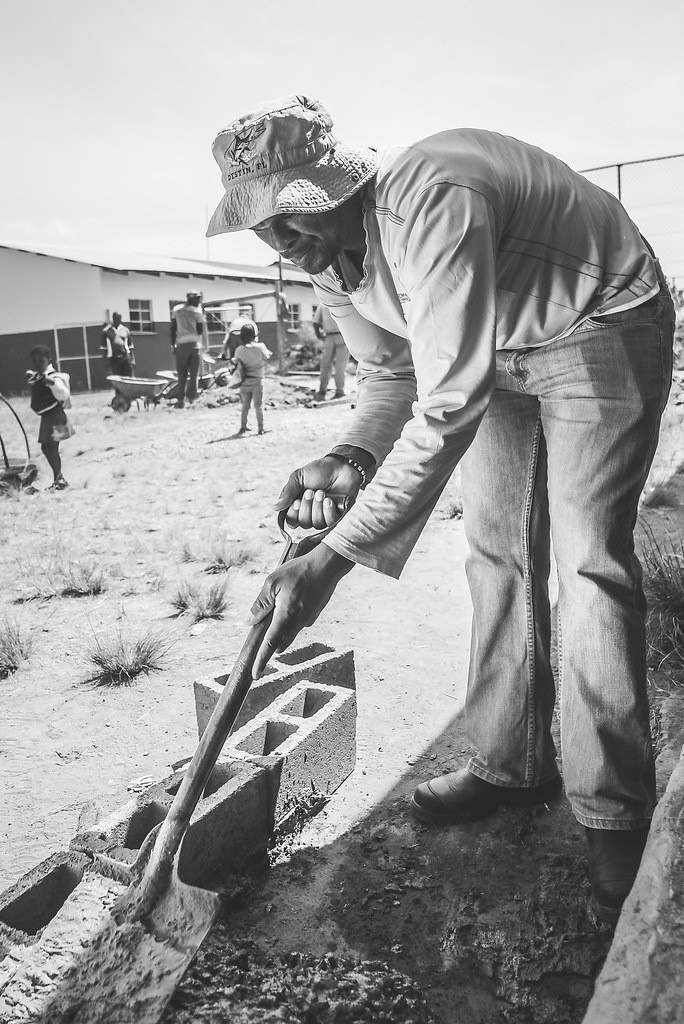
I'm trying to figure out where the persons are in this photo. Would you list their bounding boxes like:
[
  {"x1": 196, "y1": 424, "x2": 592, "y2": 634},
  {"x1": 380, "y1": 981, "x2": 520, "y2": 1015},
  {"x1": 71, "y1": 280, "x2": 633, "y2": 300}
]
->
[
  {"x1": 219, "y1": 308, "x2": 258, "y2": 372},
  {"x1": 170, "y1": 290, "x2": 203, "y2": 408},
  {"x1": 312, "y1": 302, "x2": 350, "y2": 399},
  {"x1": 205, "y1": 97, "x2": 676, "y2": 912},
  {"x1": 26, "y1": 345, "x2": 76, "y2": 491},
  {"x1": 101, "y1": 312, "x2": 136, "y2": 397},
  {"x1": 229, "y1": 324, "x2": 274, "y2": 434}
]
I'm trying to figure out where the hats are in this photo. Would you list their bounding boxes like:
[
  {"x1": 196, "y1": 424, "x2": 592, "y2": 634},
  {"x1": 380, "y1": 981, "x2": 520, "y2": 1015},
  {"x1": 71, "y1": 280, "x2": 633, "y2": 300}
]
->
[
  {"x1": 241, "y1": 324, "x2": 254, "y2": 341},
  {"x1": 186, "y1": 291, "x2": 202, "y2": 302},
  {"x1": 205, "y1": 95, "x2": 387, "y2": 238}
]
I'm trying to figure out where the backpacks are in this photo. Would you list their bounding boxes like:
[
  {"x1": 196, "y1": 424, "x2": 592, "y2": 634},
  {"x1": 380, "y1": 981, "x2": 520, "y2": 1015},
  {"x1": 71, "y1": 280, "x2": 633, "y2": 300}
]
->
[{"x1": 46, "y1": 372, "x2": 72, "y2": 401}]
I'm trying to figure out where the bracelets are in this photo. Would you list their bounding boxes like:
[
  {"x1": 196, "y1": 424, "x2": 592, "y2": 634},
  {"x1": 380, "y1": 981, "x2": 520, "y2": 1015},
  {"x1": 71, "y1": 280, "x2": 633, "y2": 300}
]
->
[{"x1": 325, "y1": 453, "x2": 365, "y2": 486}]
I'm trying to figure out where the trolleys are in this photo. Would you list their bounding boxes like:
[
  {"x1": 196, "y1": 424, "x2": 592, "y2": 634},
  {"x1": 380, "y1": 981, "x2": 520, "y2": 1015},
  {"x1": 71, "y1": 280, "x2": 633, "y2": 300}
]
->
[
  {"x1": 107, "y1": 374, "x2": 180, "y2": 414},
  {"x1": 156, "y1": 367, "x2": 230, "y2": 396}
]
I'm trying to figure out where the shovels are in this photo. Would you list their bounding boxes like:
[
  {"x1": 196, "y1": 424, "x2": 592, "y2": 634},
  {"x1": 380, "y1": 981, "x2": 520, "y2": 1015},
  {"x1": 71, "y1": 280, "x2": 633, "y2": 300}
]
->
[
  {"x1": 201, "y1": 350, "x2": 230, "y2": 364},
  {"x1": 39, "y1": 489, "x2": 355, "y2": 1024}
]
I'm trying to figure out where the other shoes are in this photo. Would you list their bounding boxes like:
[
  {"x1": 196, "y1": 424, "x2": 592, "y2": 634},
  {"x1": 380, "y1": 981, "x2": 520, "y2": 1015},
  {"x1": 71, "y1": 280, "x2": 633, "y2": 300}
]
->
[
  {"x1": 176, "y1": 401, "x2": 184, "y2": 408},
  {"x1": 585, "y1": 822, "x2": 651, "y2": 922},
  {"x1": 318, "y1": 390, "x2": 326, "y2": 396},
  {"x1": 259, "y1": 428, "x2": 271, "y2": 435},
  {"x1": 410, "y1": 766, "x2": 562, "y2": 825},
  {"x1": 335, "y1": 393, "x2": 345, "y2": 398},
  {"x1": 45, "y1": 477, "x2": 70, "y2": 493},
  {"x1": 239, "y1": 427, "x2": 252, "y2": 434}
]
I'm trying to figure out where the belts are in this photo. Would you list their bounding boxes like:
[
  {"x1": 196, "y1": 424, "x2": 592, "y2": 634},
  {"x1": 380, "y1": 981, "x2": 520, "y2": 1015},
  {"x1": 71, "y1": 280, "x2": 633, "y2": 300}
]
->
[{"x1": 326, "y1": 332, "x2": 340, "y2": 334}]
